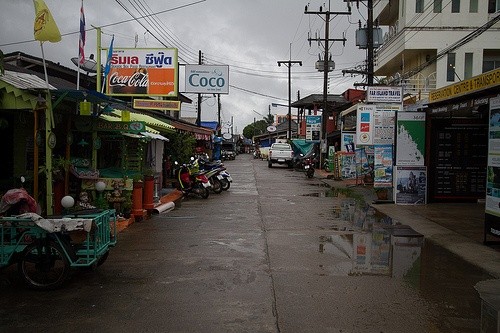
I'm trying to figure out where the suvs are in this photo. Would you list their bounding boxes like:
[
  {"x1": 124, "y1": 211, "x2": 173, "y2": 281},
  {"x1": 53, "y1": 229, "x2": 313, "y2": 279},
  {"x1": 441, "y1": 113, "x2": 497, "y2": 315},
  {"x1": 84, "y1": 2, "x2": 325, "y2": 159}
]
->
[{"x1": 221, "y1": 144, "x2": 235, "y2": 160}]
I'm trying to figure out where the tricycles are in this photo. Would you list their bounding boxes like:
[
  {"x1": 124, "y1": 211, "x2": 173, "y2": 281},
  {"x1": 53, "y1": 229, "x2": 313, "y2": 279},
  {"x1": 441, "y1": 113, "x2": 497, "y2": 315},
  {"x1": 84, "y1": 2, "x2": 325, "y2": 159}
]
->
[{"x1": 0, "y1": 210, "x2": 116, "y2": 287}]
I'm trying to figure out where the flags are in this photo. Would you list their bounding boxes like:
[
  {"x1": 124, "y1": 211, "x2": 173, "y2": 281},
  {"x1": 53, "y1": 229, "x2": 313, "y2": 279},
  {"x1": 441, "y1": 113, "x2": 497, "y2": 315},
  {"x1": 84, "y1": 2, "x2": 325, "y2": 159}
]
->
[
  {"x1": 33, "y1": 0, "x2": 62, "y2": 43},
  {"x1": 78, "y1": 7, "x2": 87, "y2": 64}
]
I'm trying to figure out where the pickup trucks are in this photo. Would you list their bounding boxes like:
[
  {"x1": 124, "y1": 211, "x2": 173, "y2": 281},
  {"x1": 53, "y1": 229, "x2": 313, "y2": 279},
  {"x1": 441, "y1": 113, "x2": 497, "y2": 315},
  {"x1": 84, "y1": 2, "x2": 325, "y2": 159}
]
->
[{"x1": 269, "y1": 143, "x2": 293, "y2": 169}]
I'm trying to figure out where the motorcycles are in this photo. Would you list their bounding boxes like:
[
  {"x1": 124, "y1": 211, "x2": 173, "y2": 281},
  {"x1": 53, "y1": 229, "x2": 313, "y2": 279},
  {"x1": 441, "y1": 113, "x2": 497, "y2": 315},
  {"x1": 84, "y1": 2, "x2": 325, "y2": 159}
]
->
[
  {"x1": 298, "y1": 152, "x2": 318, "y2": 179},
  {"x1": 191, "y1": 156, "x2": 234, "y2": 194},
  {"x1": 174, "y1": 157, "x2": 210, "y2": 198}
]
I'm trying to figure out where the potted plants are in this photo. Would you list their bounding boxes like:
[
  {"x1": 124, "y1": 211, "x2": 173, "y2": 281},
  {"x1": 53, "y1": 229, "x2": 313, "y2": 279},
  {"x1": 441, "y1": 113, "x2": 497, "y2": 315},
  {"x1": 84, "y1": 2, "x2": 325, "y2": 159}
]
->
[
  {"x1": 38, "y1": 155, "x2": 71, "y2": 193},
  {"x1": 132, "y1": 174, "x2": 144, "y2": 189},
  {"x1": 371, "y1": 186, "x2": 394, "y2": 200},
  {"x1": 142, "y1": 166, "x2": 156, "y2": 181}
]
